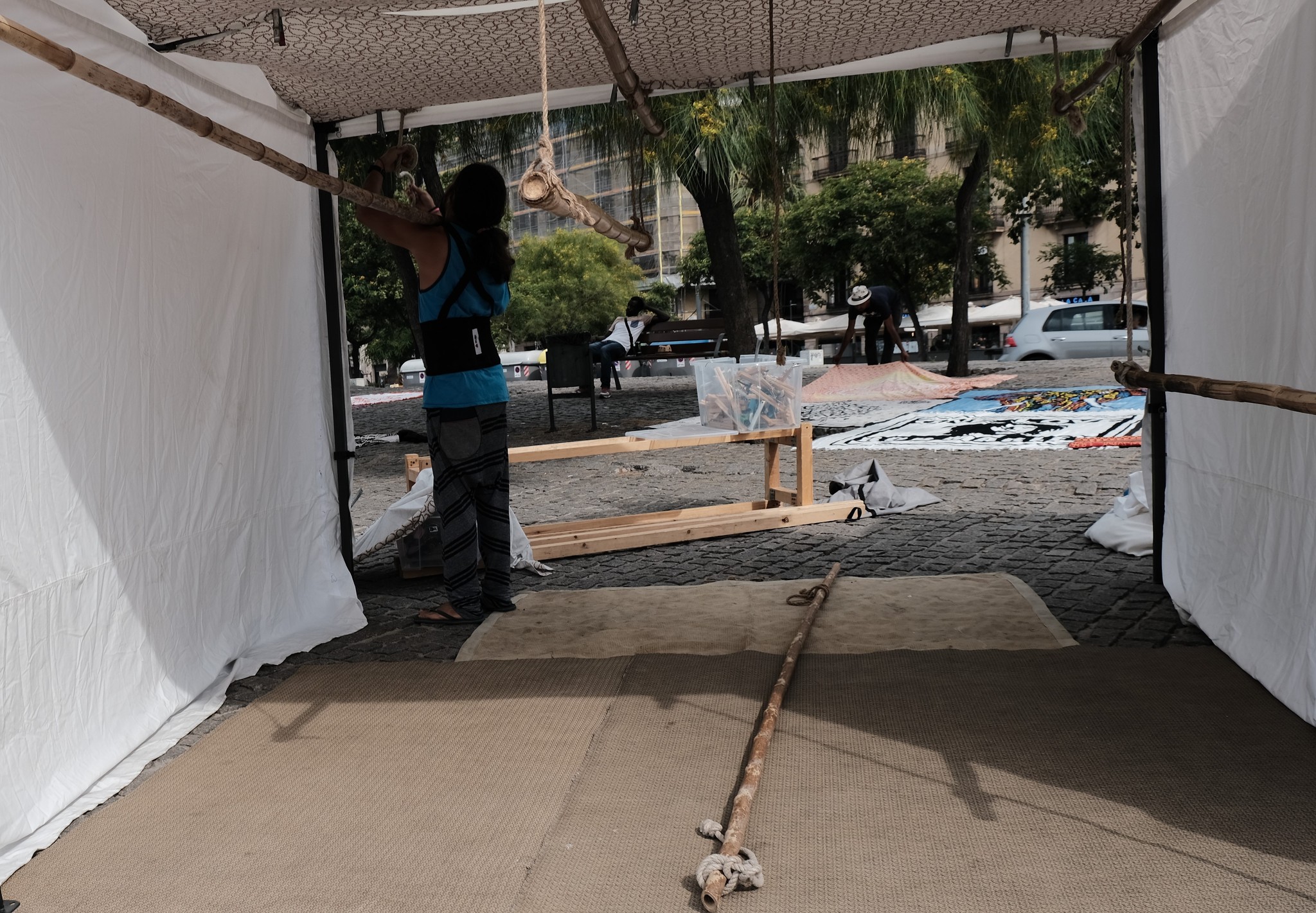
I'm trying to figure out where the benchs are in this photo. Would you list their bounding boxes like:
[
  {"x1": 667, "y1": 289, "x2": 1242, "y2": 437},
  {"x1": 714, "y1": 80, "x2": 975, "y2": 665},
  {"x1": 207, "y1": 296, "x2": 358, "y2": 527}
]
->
[{"x1": 610, "y1": 315, "x2": 740, "y2": 391}]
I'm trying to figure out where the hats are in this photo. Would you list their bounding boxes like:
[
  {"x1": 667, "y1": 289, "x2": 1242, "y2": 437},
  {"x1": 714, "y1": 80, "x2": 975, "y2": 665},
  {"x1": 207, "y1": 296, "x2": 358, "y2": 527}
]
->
[{"x1": 846, "y1": 285, "x2": 872, "y2": 306}]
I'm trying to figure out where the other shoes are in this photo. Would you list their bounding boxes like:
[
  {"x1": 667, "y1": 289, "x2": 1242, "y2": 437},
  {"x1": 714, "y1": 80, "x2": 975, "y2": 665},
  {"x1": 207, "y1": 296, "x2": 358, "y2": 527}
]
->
[
  {"x1": 599, "y1": 387, "x2": 612, "y2": 398},
  {"x1": 569, "y1": 387, "x2": 595, "y2": 396}
]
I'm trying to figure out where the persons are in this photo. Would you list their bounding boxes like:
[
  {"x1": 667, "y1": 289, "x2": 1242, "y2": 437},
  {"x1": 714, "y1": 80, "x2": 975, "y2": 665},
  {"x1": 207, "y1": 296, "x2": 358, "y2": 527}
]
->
[
  {"x1": 832, "y1": 285, "x2": 910, "y2": 366},
  {"x1": 355, "y1": 146, "x2": 515, "y2": 626},
  {"x1": 570, "y1": 296, "x2": 671, "y2": 399},
  {"x1": 1123, "y1": 313, "x2": 1142, "y2": 330}
]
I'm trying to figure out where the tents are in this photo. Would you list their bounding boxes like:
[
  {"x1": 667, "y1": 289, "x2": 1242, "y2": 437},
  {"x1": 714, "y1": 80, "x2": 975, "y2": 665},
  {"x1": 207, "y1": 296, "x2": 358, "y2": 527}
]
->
[{"x1": 753, "y1": 293, "x2": 1071, "y2": 357}]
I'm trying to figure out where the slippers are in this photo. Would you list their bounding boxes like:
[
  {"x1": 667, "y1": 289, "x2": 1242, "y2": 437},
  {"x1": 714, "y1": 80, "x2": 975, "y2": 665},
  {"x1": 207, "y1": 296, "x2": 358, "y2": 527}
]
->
[
  {"x1": 413, "y1": 603, "x2": 483, "y2": 626},
  {"x1": 485, "y1": 603, "x2": 516, "y2": 612}
]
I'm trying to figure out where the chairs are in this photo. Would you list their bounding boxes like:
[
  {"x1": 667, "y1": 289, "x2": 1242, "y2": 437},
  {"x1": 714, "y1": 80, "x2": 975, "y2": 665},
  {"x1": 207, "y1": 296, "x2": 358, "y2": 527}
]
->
[{"x1": 543, "y1": 331, "x2": 599, "y2": 434}]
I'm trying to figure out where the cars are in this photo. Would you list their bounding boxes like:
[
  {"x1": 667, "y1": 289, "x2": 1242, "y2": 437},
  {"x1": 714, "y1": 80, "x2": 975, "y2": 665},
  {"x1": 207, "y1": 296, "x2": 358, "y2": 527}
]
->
[{"x1": 998, "y1": 298, "x2": 1152, "y2": 361}]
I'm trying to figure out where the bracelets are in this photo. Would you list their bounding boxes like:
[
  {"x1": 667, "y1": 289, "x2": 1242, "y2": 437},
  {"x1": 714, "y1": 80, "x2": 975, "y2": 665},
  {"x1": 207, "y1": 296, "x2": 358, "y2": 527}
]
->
[
  {"x1": 367, "y1": 164, "x2": 386, "y2": 178},
  {"x1": 646, "y1": 305, "x2": 651, "y2": 312},
  {"x1": 427, "y1": 206, "x2": 440, "y2": 214},
  {"x1": 378, "y1": 158, "x2": 386, "y2": 174}
]
